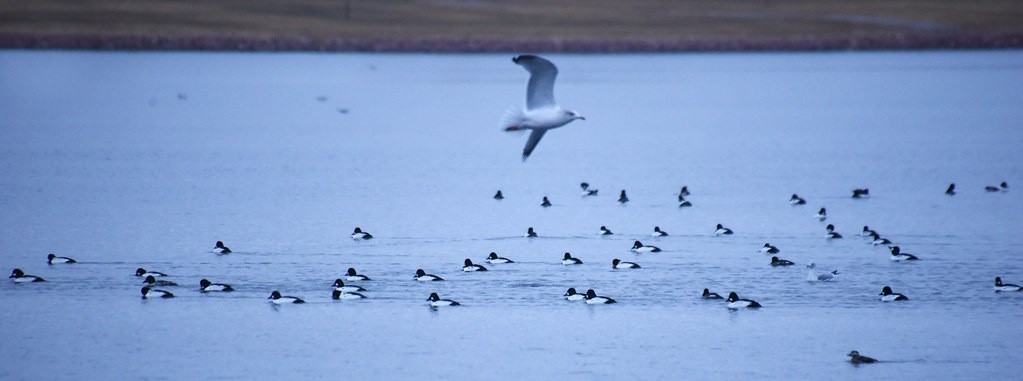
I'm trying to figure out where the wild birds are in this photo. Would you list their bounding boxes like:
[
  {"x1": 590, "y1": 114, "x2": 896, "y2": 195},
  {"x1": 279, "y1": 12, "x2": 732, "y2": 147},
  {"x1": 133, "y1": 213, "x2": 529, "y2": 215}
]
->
[
  {"x1": 725, "y1": 292, "x2": 762, "y2": 308},
  {"x1": 9, "y1": 269, "x2": 47, "y2": 282},
  {"x1": 993, "y1": 276, "x2": 1023, "y2": 292},
  {"x1": 332, "y1": 288, "x2": 366, "y2": 299},
  {"x1": 211, "y1": 241, "x2": 233, "y2": 255},
  {"x1": 426, "y1": 292, "x2": 461, "y2": 306},
  {"x1": 878, "y1": 286, "x2": 909, "y2": 302},
  {"x1": 267, "y1": 291, "x2": 306, "y2": 303},
  {"x1": 499, "y1": 53, "x2": 585, "y2": 163},
  {"x1": 563, "y1": 288, "x2": 587, "y2": 301},
  {"x1": 47, "y1": 253, "x2": 77, "y2": 265},
  {"x1": 141, "y1": 275, "x2": 178, "y2": 286},
  {"x1": 582, "y1": 289, "x2": 617, "y2": 305},
  {"x1": 331, "y1": 278, "x2": 366, "y2": 292},
  {"x1": 345, "y1": 182, "x2": 921, "y2": 284},
  {"x1": 846, "y1": 351, "x2": 878, "y2": 362},
  {"x1": 135, "y1": 268, "x2": 168, "y2": 277},
  {"x1": 199, "y1": 279, "x2": 235, "y2": 291},
  {"x1": 701, "y1": 288, "x2": 724, "y2": 299},
  {"x1": 141, "y1": 287, "x2": 175, "y2": 297}
]
